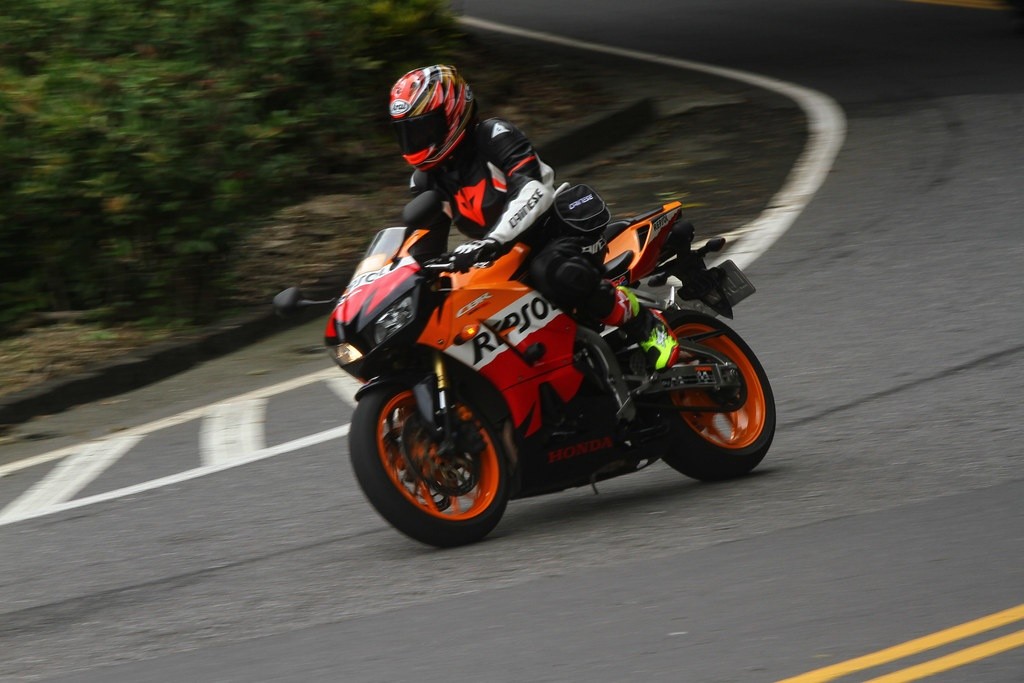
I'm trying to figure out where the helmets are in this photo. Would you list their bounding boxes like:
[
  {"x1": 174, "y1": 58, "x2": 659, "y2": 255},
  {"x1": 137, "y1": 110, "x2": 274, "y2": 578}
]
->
[{"x1": 389, "y1": 63, "x2": 473, "y2": 171}]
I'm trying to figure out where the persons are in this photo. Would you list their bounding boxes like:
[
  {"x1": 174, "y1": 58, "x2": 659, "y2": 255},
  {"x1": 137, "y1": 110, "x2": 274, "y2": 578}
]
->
[{"x1": 389, "y1": 64, "x2": 679, "y2": 374}]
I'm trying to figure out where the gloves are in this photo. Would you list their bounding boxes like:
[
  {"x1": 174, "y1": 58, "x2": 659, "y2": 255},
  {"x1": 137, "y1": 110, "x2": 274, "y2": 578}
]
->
[{"x1": 450, "y1": 238, "x2": 500, "y2": 273}]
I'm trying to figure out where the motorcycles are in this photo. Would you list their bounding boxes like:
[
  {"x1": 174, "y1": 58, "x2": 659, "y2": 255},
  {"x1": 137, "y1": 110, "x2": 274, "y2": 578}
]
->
[{"x1": 323, "y1": 188, "x2": 778, "y2": 548}]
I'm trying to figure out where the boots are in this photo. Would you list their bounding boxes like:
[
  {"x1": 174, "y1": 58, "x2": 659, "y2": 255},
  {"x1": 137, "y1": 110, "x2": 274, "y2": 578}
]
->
[{"x1": 597, "y1": 281, "x2": 680, "y2": 372}]
